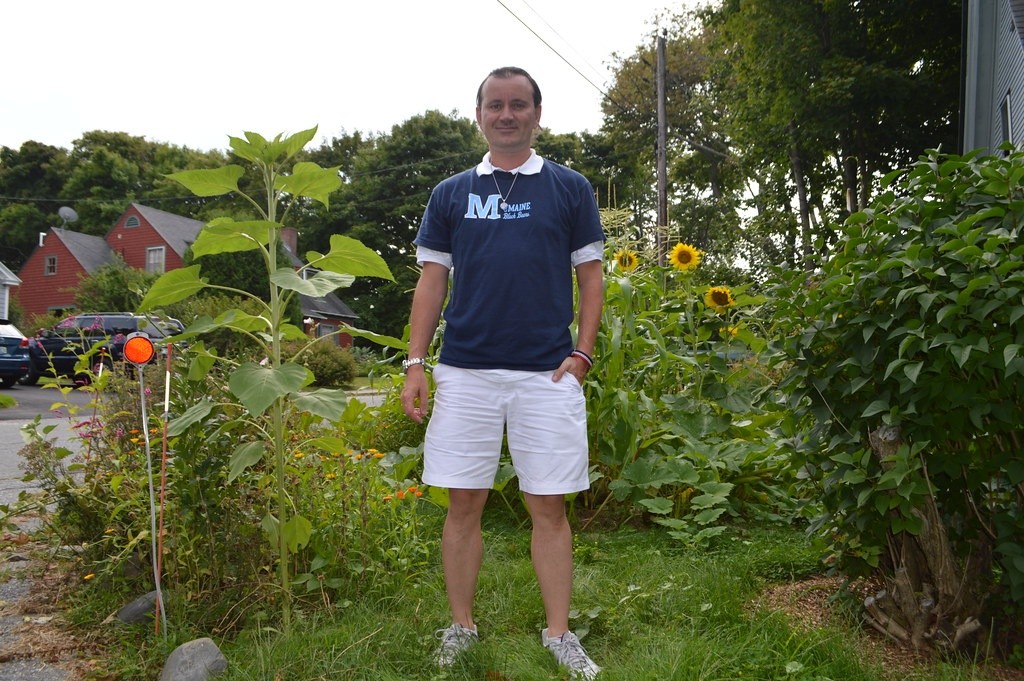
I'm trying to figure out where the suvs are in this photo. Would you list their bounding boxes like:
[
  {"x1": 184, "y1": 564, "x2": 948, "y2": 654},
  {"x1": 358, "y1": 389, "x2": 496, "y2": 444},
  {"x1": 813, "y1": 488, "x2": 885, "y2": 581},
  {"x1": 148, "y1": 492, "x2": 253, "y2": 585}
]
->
[
  {"x1": 19, "y1": 311, "x2": 189, "y2": 387},
  {"x1": 1, "y1": 318, "x2": 30, "y2": 388}
]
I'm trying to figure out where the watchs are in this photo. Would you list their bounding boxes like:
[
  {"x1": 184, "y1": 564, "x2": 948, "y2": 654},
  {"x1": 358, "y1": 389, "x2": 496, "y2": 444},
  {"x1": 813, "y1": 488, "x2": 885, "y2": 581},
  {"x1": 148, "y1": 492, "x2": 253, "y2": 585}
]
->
[{"x1": 402, "y1": 357, "x2": 425, "y2": 374}]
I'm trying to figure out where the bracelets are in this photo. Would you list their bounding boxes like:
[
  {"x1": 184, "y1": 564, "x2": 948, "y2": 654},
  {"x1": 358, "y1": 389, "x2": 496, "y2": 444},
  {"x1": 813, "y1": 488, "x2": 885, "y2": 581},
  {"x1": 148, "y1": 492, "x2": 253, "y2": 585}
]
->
[{"x1": 571, "y1": 350, "x2": 593, "y2": 367}]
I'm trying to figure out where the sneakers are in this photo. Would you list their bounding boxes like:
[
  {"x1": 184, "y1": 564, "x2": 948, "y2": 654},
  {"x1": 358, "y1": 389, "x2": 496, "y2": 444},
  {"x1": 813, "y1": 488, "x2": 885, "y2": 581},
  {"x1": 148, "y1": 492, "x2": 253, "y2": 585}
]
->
[
  {"x1": 543, "y1": 628, "x2": 601, "y2": 679},
  {"x1": 432, "y1": 622, "x2": 478, "y2": 674}
]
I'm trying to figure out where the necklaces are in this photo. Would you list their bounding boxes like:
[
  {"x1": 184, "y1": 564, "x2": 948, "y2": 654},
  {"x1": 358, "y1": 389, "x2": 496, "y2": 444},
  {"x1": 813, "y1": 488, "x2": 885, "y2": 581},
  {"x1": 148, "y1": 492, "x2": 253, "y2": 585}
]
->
[{"x1": 491, "y1": 164, "x2": 521, "y2": 210}]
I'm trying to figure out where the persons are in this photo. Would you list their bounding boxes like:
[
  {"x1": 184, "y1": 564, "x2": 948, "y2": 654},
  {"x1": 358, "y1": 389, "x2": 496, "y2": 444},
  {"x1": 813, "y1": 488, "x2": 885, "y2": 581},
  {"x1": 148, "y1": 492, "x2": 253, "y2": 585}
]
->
[{"x1": 399, "y1": 66, "x2": 607, "y2": 681}]
